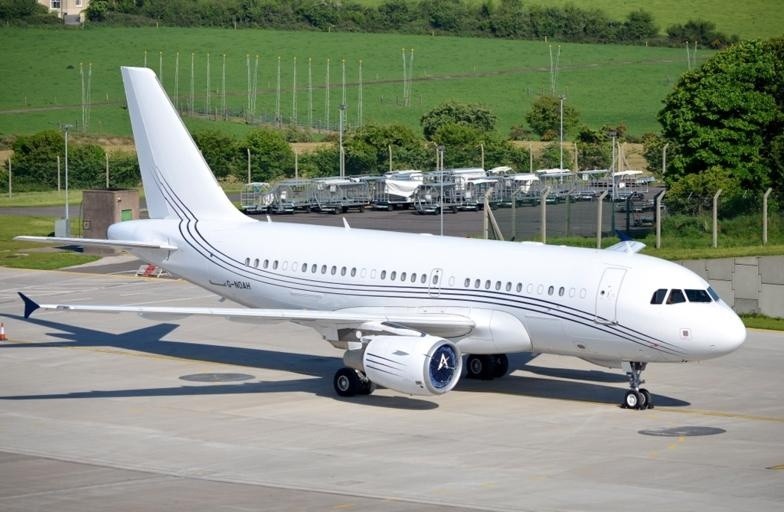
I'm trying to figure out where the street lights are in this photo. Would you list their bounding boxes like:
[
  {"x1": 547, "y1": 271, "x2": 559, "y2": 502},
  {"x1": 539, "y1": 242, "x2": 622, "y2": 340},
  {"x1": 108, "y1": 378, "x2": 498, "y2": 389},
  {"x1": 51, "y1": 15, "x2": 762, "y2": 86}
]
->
[
  {"x1": 558, "y1": 94, "x2": 568, "y2": 185},
  {"x1": 335, "y1": 101, "x2": 352, "y2": 178},
  {"x1": 64, "y1": 121, "x2": 76, "y2": 218},
  {"x1": 606, "y1": 125, "x2": 619, "y2": 204},
  {"x1": 435, "y1": 142, "x2": 448, "y2": 236}
]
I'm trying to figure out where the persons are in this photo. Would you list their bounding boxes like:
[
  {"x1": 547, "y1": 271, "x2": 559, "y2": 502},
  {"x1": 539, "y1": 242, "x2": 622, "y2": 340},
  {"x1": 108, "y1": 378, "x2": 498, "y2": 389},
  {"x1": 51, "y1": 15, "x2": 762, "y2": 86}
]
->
[{"x1": 666, "y1": 294, "x2": 673, "y2": 304}]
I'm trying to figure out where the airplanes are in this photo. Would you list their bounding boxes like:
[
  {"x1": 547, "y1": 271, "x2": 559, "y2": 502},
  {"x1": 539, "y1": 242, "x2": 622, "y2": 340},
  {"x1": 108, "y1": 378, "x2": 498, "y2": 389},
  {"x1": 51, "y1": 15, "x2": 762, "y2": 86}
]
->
[{"x1": 9, "y1": 64, "x2": 754, "y2": 412}]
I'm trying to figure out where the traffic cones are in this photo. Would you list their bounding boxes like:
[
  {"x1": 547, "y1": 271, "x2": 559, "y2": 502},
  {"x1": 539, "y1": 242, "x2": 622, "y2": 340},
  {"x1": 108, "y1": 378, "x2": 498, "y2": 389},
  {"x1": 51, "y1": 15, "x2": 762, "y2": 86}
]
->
[{"x1": 0, "y1": 320, "x2": 9, "y2": 341}]
